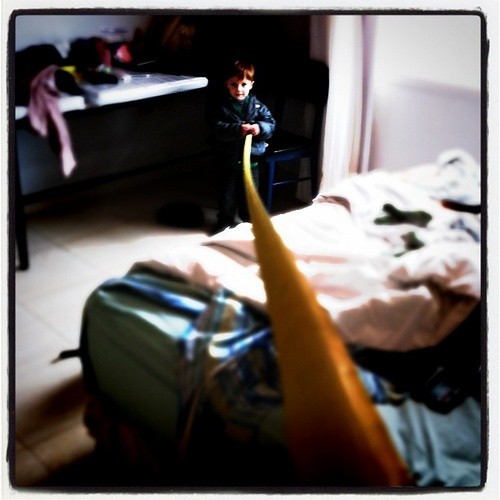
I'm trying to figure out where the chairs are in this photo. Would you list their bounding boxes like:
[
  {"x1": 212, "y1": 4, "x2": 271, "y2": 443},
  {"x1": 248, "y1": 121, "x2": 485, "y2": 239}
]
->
[{"x1": 257, "y1": 57, "x2": 330, "y2": 215}]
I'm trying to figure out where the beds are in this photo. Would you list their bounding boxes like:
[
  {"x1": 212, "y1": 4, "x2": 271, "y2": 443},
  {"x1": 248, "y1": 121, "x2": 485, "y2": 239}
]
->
[{"x1": 74, "y1": 150, "x2": 486, "y2": 489}]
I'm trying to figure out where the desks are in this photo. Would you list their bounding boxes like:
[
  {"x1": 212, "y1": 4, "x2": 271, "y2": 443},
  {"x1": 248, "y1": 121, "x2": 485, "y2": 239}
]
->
[{"x1": 15, "y1": 70, "x2": 210, "y2": 271}]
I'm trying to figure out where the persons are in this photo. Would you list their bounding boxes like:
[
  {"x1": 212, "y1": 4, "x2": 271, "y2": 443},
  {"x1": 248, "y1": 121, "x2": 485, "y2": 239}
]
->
[{"x1": 210, "y1": 58, "x2": 278, "y2": 238}]
